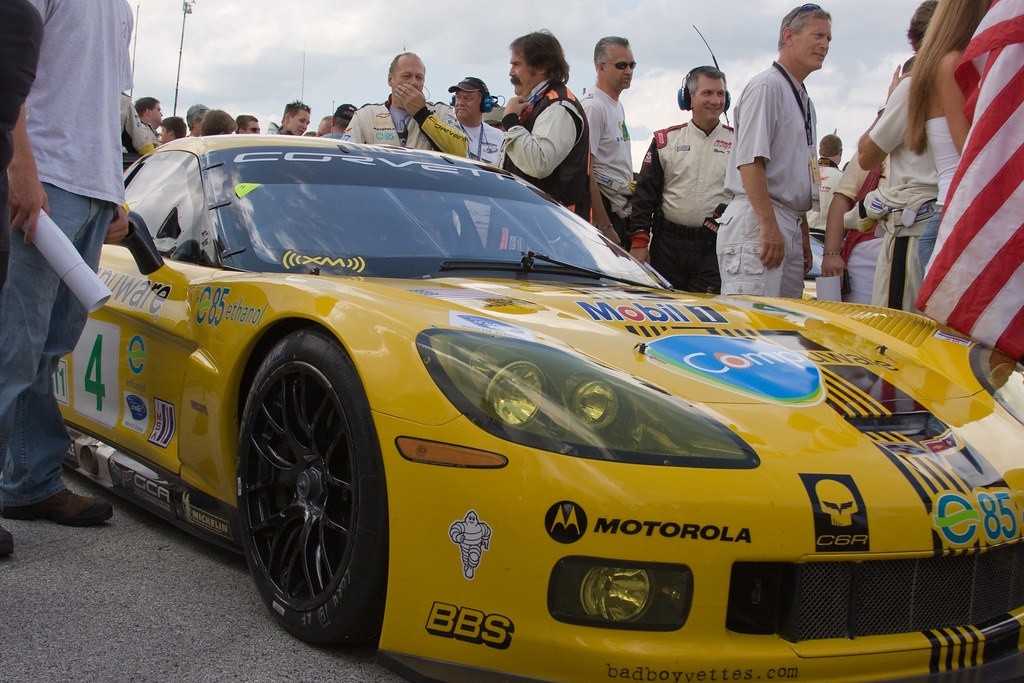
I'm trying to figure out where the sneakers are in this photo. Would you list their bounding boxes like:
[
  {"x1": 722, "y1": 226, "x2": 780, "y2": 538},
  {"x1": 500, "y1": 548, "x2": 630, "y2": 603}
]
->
[{"x1": 4, "y1": 487, "x2": 113, "y2": 528}]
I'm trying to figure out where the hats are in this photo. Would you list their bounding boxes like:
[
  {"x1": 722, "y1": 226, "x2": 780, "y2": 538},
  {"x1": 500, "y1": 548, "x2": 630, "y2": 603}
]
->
[
  {"x1": 448, "y1": 77, "x2": 489, "y2": 96},
  {"x1": 334, "y1": 104, "x2": 357, "y2": 119}
]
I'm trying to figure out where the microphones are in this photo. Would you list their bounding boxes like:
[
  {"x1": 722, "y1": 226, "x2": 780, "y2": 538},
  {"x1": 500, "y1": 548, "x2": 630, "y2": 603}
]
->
[{"x1": 693, "y1": 25, "x2": 718, "y2": 70}]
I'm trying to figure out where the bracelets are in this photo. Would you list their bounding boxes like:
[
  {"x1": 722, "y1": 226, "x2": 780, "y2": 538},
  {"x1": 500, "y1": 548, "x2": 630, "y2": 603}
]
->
[
  {"x1": 822, "y1": 251, "x2": 841, "y2": 255},
  {"x1": 599, "y1": 224, "x2": 613, "y2": 230},
  {"x1": 877, "y1": 105, "x2": 886, "y2": 113}
]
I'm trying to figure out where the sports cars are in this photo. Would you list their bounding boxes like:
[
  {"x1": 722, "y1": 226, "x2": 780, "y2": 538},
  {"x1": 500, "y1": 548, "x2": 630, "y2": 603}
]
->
[{"x1": 52, "y1": 127, "x2": 1023, "y2": 683}]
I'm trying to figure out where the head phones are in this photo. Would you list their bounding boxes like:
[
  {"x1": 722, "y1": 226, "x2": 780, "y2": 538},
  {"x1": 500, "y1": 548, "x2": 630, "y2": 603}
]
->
[
  {"x1": 451, "y1": 94, "x2": 498, "y2": 113},
  {"x1": 678, "y1": 66, "x2": 731, "y2": 113}
]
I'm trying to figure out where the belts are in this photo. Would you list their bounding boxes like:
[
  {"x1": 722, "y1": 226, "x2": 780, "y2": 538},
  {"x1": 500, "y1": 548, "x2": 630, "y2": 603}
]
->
[{"x1": 656, "y1": 219, "x2": 716, "y2": 240}]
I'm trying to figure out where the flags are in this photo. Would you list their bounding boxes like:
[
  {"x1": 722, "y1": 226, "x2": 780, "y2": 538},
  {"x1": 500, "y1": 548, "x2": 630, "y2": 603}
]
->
[{"x1": 916, "y1": 0, "x2": 1024, "y2": 361}]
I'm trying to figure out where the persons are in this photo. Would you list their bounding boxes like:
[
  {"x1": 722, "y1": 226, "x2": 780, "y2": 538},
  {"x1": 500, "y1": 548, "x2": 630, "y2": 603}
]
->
[
  {"x1": 121, "y1": 89, "x2": 357, "y2": 154},
  {"x1": 579, "y1": 36, "x2": 637, "y2": 246},
  {"x1": 629, "y1": 66, "x2": 734, "y2": 295},
  {"x1": 806, "y1": 0, "x2": 993, "y2": 318},
  {"x1": 448, "y1": 77, "x2": 504, "y2": 249},
  {"x1": 340, "y1": 53, "x2": 469, "y2": 158},
  {"x1": 0, "y1": 0, "x2": 135, "y2": 556},
  {"x1": 498, "y1": 28, "x2": 593, "y2": 267},
  {"x1": 715, "y1": 4, "x2": 832, "y2": 299}
]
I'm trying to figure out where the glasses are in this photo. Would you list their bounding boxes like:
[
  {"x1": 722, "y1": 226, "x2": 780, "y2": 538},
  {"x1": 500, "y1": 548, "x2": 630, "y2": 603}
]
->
[
  {"x1": 286, "y1": 103, "x2": 311, "y2": 115},
  {"x1": 598, "y1": 61, "x2": 636, "y2": 70},
  {"x1": 243, "y1": 128, "x2": 260, "y2": 133},
  {"x1": 787, "y1": 3, "x2": 822, "y2": 26}
]
